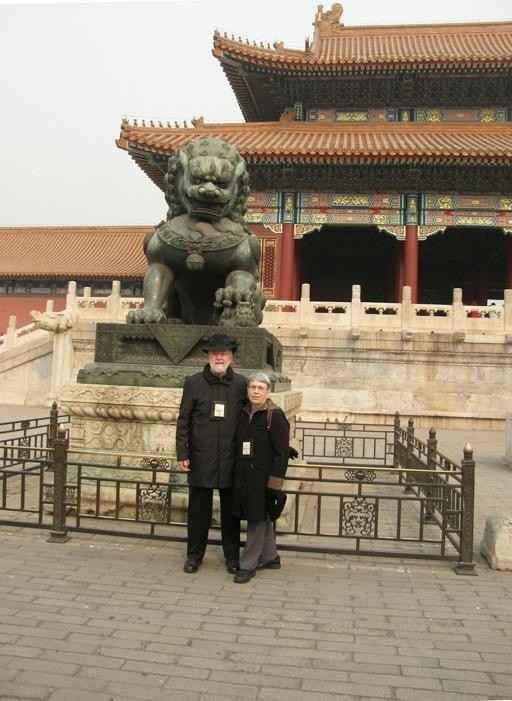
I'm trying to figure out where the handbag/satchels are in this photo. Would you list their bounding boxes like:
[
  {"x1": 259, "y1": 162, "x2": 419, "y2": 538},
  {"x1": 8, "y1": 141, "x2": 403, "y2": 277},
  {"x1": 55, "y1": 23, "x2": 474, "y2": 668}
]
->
[{"x1": 267, "y1": 490, "x2": 288, "y2": 521}]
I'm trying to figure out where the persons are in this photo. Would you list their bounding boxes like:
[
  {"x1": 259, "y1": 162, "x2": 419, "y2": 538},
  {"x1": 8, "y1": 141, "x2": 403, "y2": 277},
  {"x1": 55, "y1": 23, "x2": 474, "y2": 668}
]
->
[
  {"x1": 468, "y1": 300, "x2": 482, "y2": 319},
  {"x1": 484, "y1": 301, "x2": 498, "y2": 318},
  {"x1": 173, "y1": 332, "x2": 301, "y2": 577},
  {"x1": 230, "y1": 371, "x2": 291, "y2": 584}
]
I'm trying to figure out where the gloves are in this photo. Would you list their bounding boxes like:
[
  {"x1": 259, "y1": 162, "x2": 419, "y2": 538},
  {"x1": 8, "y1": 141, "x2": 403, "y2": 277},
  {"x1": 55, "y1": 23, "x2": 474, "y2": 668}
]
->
[
  {"x1": 288, "y1": 445, "x2": 298, "y2": 460},
  {"x1": 266, "y1": 488, "x2": 281, "y2": 499}
]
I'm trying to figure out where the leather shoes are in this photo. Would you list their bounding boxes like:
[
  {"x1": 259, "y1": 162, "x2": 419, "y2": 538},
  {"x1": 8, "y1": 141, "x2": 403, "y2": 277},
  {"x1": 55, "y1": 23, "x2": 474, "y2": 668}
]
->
[
  {"x1": 224, "y1": 559, "x2": 240, "y2": 574},
  {"x1": 234, "y1": 570, "x2": 257, "y2": 583},
  {"x1": 257, "y1": 556, "x2": 282, "y2": 570},
  {"x1": 182, "y1": 558, "x2": 204, "y2": 573}
]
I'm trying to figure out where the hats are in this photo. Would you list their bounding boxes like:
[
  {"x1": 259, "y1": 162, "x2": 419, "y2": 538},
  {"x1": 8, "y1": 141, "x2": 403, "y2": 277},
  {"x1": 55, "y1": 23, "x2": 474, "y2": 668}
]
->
[{"x1": 200, "y1": 333, "x2": 241, "y2": 356}]
